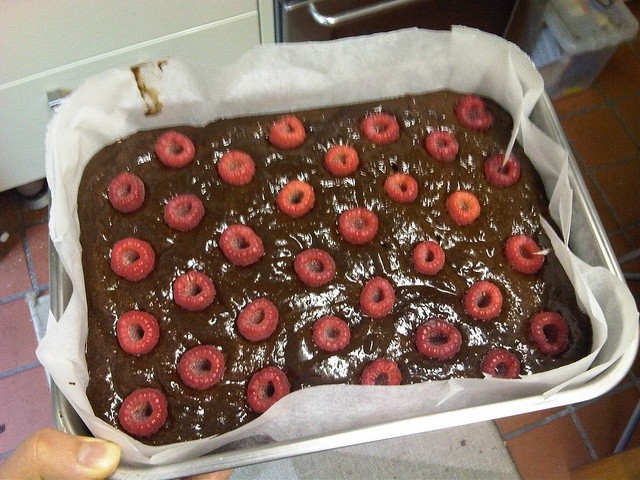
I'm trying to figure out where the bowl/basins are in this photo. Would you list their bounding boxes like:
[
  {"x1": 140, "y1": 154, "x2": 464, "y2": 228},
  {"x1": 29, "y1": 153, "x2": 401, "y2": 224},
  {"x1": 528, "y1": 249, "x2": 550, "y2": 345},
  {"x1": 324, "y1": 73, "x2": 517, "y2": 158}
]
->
[{"x1": 47, "y1": 88, "x2": 640, "y2": 479}]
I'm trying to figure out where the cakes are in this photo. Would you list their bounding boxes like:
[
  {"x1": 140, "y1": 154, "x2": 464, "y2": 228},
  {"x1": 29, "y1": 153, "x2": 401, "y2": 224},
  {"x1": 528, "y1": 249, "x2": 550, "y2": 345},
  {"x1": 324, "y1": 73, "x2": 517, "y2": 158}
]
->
[{"x1": 75, "y1": 87, "x2": 593, "y2": 447}]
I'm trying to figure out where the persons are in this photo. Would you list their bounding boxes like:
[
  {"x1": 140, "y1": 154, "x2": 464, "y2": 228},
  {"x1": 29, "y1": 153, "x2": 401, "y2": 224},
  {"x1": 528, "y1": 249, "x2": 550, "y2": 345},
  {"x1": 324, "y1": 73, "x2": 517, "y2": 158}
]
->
[{"x1": 0, "y1": 429, "x2": 232, "y2": 480}]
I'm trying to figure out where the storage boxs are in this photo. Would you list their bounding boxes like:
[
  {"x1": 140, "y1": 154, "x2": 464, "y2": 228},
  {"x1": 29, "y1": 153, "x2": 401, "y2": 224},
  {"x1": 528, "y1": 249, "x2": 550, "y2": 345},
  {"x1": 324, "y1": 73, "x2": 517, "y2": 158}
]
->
[{"x1": 532, "y1": 1, "x2": 639, "y2": 101}]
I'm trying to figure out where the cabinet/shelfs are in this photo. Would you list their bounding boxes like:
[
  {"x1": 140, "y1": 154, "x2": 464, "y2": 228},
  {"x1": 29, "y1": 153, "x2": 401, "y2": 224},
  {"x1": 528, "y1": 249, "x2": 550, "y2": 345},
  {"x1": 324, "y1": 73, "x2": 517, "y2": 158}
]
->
[
  {"x1": 275, "y1": 0, "x2": 528, "y2": 48},
  {"x1": 0, "y1": 1, "x2": 277, "y2": 199}
]
